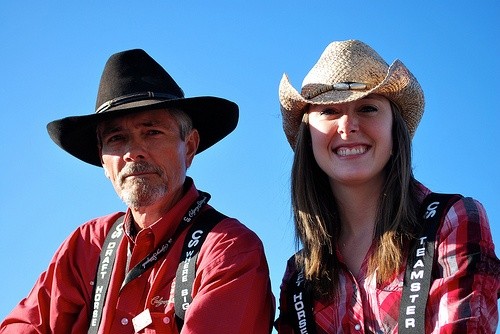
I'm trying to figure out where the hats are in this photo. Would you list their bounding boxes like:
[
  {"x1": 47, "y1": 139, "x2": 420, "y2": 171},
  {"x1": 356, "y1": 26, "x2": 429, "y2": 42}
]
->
[
  {"x1": 46, "y1": 49, "x2": 240, "y2": 168},
  {"x1": 278, "y1": 39, "x2": 426, "y2": 153}
]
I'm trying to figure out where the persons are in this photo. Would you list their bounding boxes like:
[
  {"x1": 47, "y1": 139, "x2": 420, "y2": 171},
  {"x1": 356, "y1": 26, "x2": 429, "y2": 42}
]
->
[
  {"x1": 1, "y1": 46, "x2": 277, "y2": 334},
  {"x1": 273, "y1": 40, "x2": 500, "y2": 334}
]
